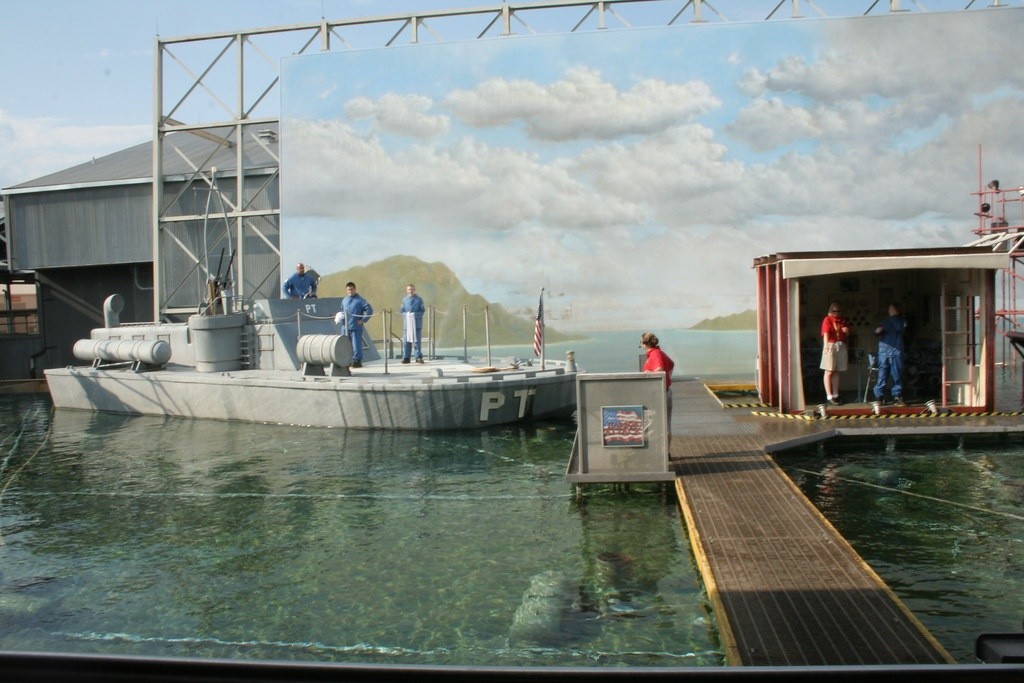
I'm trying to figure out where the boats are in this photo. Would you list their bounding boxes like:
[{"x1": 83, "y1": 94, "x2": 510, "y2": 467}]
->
[{"x1": 43, "y1": 290, "x2": 586, "y2": 430}]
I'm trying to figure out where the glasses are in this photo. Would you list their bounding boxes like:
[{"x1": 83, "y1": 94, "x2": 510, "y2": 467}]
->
[{"x1": 832, "y1": 310, "x2": 841, "y2": 313}]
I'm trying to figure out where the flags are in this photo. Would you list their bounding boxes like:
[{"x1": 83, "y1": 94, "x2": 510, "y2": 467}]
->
[{"x1": 534, "y1": 291, "x2": 544, "y2": 357}]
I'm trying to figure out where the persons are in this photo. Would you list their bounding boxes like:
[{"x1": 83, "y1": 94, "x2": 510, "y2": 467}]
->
[
  {"x1": 397, "y1": 285, "x2": 425, "y2": 364},
  {"x1": 282, "y1": 262, "x2": 318, "y2": 300},
  {"x1": 873, "y1": 303, "x2": 908, "y2": 408},
  {"x1": 339, "y1": 282, "x2": 372, "y2": 367},
  {"x1": 820, "y1": 302, "x2": 853, "y2": 405},
  {"x1": 640, "y1": 331, "x2": 674, "y2": 457}
]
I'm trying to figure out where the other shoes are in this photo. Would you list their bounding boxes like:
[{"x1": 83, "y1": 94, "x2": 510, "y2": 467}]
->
[
  {"x1": 894, "y1": 397, "x2": 907, "y2": 407},
  {"x1": 416, "y1": 357, "x2": 424, "y2": 364},
  {"x1": 402, "y1": 358, "x2": 410, "y2": 364},
  {"x1": 829, "y1": 397, "x2": 844, "y2": 407},
  {"x1": 873, "y1": 397, "x2": 883, "y2": 406},
  {"x1": 351, "y1": 362, "x2": 362, "y2": 367}
]
